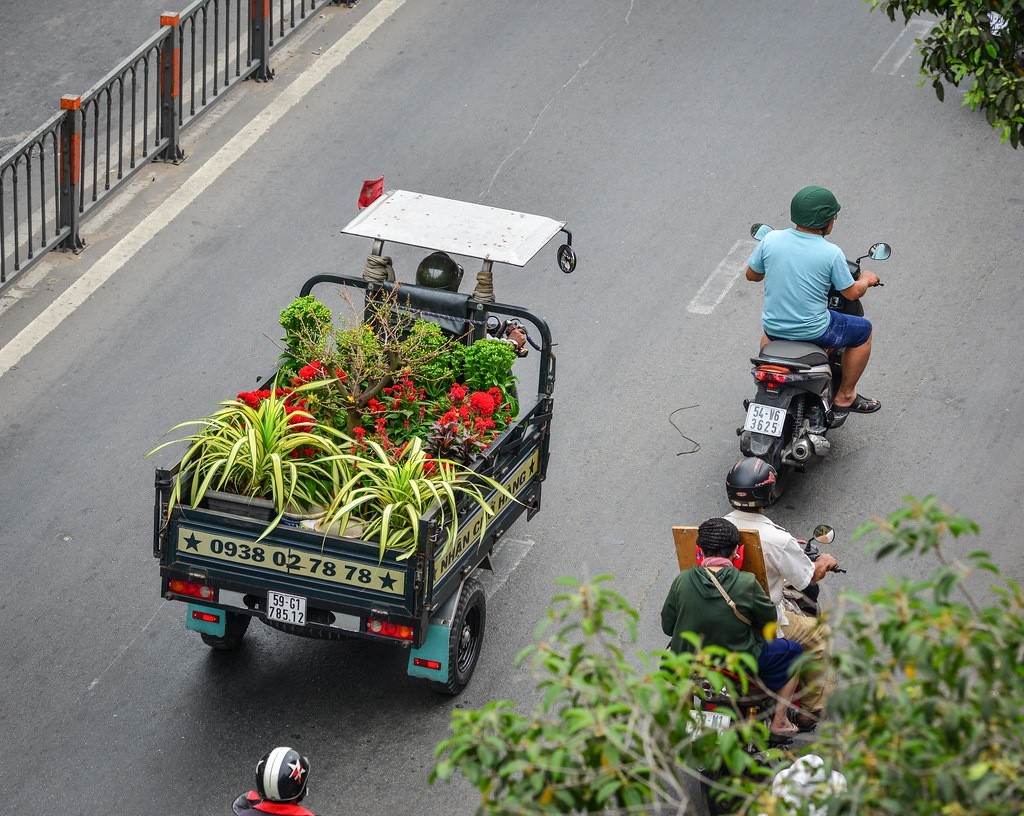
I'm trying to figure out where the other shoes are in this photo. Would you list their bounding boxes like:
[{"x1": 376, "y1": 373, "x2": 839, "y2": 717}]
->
[
  {"x1": 769, "y1": 723, "x2": 799, "y2": 736},
  {"x1": 802, "y1": 711, "x2": 829, "y2": 726}
]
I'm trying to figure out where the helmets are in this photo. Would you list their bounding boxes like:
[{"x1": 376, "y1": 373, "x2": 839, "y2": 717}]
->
[
  {"x1": 417, "y1": 250, "x2": 466, "y2": 291},
  {"x1": 791, "y1": 185, "x2": 841, "y2": 229},
  {"x1": 726, "y1": 457, "x2": 776, "y2": 507},
  {"x1": 256, "y1": 746, "x2": 311, "y2": 803}
]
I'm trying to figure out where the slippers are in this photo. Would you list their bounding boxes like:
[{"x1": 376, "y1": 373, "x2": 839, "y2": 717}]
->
[{"x1": 833, "y1": 394, "x2": 881, "y2": 414}]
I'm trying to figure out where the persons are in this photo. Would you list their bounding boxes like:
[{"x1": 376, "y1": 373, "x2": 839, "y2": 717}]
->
[
  {"x1": 661, "y1": 456, "x2": 836, "y2": 736},
  {"x1": 746, "y1": 186, "x2": 882, "y2": 414},
  {"x1": 231, "y1": 747, "x2": 320, "y2": 816},
  {"x1": 415, "y1": 251, "x2": 526, "y2": 416}
]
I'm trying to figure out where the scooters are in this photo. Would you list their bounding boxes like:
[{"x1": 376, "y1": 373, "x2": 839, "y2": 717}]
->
[
  {"x1": 684, "y1": 523, "x2": 846, "y2": 816},
  {"x1": 735, "y1": 222, "x2": 892, "y2": 508}
]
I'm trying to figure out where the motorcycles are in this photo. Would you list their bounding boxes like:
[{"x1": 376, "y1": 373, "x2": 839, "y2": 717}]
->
[{"x1": 151, "y1": 188, "x2": 576, "y2": 698}]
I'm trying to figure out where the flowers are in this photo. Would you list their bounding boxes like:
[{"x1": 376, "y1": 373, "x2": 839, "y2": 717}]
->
[{"x1": 230, "y1": 360, "x2": 515, "y2": 478}]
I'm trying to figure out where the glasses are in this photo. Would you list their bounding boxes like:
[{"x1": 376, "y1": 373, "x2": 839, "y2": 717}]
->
[{"x1": 833, "y1": 213, "x2": 838, "y2": 219}]
